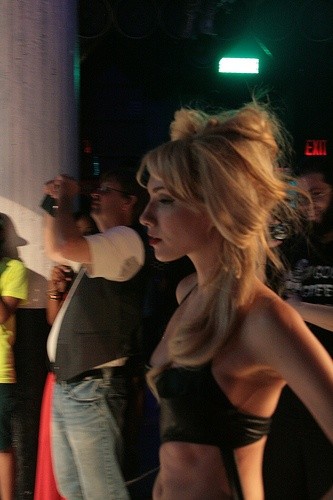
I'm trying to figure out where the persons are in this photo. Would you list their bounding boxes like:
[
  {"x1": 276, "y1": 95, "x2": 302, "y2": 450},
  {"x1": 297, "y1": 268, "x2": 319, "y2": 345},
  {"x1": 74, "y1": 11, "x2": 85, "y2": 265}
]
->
[
  {"x1": 28, "y1": 167, "x2": 148, "y2": 500},
  {"x1": 140, "y1": 102, "x2": 333, "y2": 500},
  {"x1": 0, "y1": 214, "x2": 29, "y2": 500}
]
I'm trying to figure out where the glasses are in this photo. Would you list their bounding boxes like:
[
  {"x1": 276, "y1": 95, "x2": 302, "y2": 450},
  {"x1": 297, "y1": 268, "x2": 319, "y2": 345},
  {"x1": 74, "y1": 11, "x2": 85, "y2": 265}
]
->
[{"x1": 99, "y1": 184, "x2": 130, "y2": 198}]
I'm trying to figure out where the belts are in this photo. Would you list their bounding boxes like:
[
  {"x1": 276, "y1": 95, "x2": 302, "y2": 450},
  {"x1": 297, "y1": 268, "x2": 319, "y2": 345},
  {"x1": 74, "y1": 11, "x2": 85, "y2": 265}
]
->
[{"x1": 66, "y1": 368, "x2": 106, "y2": 386}]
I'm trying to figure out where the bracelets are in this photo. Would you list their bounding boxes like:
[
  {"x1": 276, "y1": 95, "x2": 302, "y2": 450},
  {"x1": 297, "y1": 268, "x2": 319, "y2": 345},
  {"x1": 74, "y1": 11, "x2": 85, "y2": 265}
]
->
[{"x1": 48, "y1": 292, "x2": 63, "y2": 300}]
